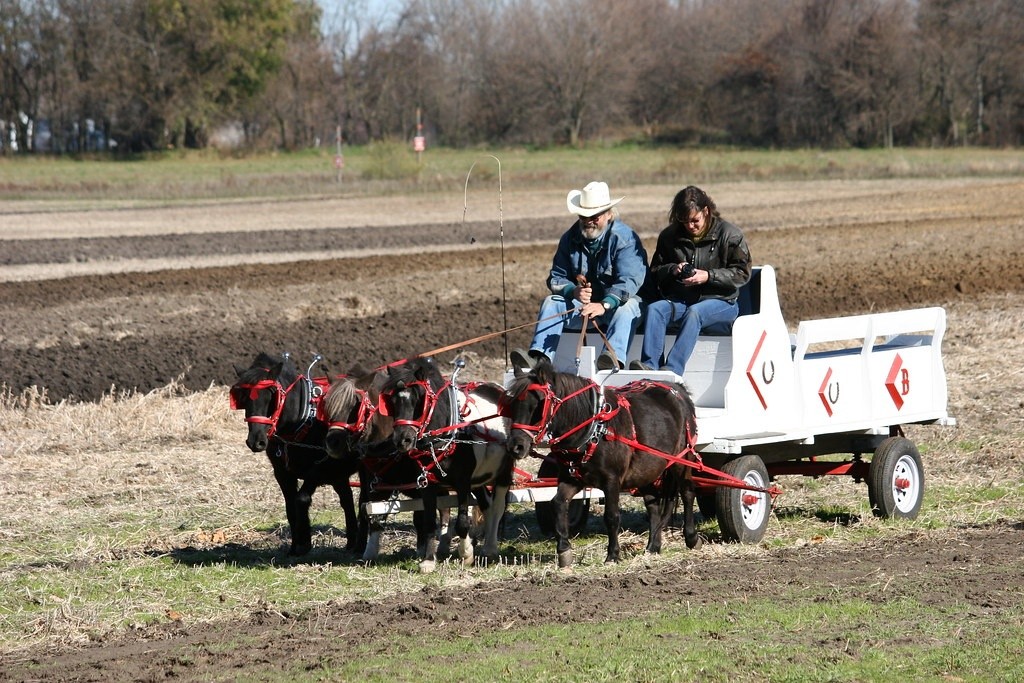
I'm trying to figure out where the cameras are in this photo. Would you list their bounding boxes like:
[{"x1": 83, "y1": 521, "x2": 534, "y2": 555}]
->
[{"x1": 676, "y1": 264, "x2": 697, "y2": 283}]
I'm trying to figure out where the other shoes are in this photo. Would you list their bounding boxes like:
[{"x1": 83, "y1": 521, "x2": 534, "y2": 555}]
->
[
  {"x1": 630, "y1": 359, "x2": 667, "y2": 371},
  {"x1": 509, "y1": 347, "x2": 548, "y2": 368},
  {"x1": 597, "y1": 353, "x2": 615, "y2": 370}
]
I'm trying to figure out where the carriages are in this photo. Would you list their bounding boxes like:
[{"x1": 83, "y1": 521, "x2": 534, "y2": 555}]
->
[{"x1": 230, "y1": 265, "x2": 948, "y2": 574}]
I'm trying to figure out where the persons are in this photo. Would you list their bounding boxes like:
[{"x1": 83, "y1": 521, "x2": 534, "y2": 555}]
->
[
  {"x1": 510, "y1": 181, "x2": 649, "y2": 370},
  {"x1": 628, "y1": 186, "x2": 753, "y2": 376}
]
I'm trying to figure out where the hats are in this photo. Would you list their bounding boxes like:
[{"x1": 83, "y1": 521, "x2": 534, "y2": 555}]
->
[{"x1": 567, "y1": 179, "x2": 626, "y2": 217}]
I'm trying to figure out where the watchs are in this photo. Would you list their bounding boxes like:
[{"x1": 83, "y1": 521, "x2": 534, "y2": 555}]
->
[{"x1": 600, "y1": 301, "x2": 611, "y2": 313}]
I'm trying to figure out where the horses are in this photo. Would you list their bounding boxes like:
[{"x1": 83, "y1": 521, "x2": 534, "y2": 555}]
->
[{"x1": 227, "y1": 350, "x2": 704, "y2": 578}]
